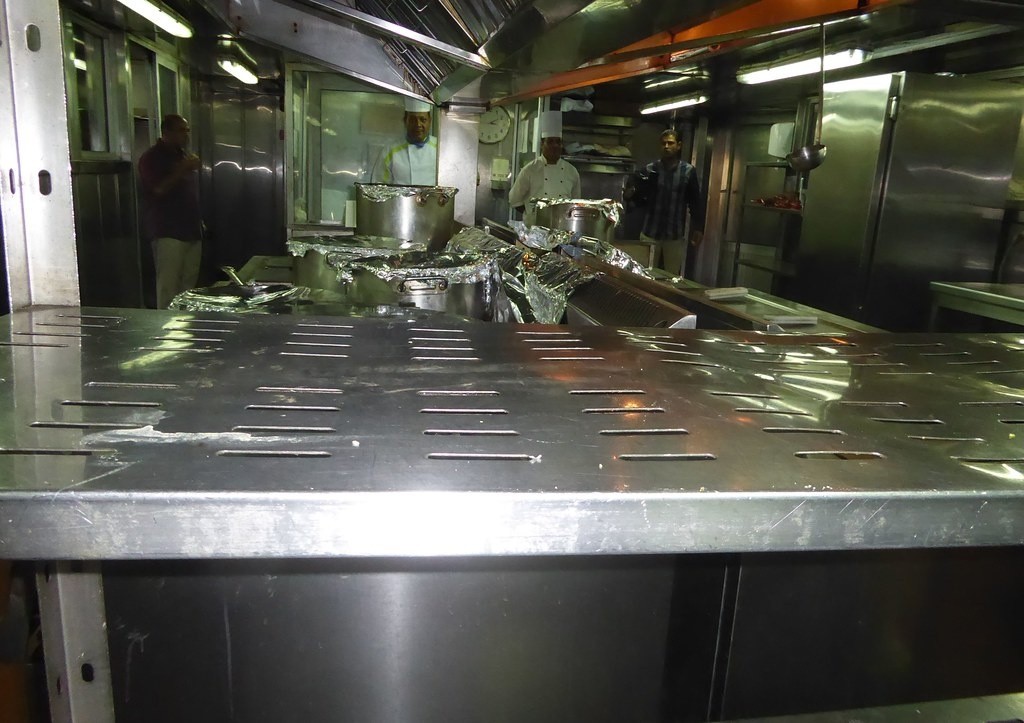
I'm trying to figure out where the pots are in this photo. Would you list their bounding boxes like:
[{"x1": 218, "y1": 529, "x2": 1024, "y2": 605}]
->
[
  {"x1": 292, "y1": 235, "x2": 490, "y2": 320},
  {"x1": 354, "y1": 182, "x2": 459, "y2": 234},
  {"x1": 529, "y1": 197, "x2": 615, "y2": 247}
]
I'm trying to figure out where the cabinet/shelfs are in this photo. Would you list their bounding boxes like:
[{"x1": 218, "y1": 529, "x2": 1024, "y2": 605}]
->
[{"x1": 732, "y1": 161, "x2": 806, "y2": 296}]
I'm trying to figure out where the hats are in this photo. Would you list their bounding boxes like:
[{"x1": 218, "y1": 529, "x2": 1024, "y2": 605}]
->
[
  {"x1": 404, "y1": 95, "x2": 430, "y2": 113},
  {"x1": 540, "y1": 111, "x2": 563, "y2": 139}
]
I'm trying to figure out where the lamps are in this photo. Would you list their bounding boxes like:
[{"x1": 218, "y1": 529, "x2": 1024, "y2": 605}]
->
[
  {"x1": 639, "y1": 88, "x2": 710, "y2": 115},
  {"x1": 215, "y1": 34, "x2": 260, "y2": 84},
  {"x1": 736, "y1": 36, "x2": 874, "y2": 85},
  {"x1": 112, "y1": 0, "x2": 195, "y2": 39}
]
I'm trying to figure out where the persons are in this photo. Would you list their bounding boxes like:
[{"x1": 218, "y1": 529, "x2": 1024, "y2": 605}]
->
[
  {"x1": 638, "y1": 129, "x2": 705, "y2": 275},
  {"x1": 508, "y1": 110, "x2": 582, "y2": 228},
  {"x1": 370, "y1": 95, "x2": 481, "y2": 186},
  {"x1": 137, "y1": 113, "x2": 204, "y2": 310}
]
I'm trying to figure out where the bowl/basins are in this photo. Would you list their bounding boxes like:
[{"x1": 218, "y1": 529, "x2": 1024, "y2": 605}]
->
[{"x1": 786, "y1": 144, "x2": 827, "y2": 170}]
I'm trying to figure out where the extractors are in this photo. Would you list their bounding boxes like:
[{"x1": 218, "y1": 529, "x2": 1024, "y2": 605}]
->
[{"x1": 176, "y1": 0, "x2": 597, "y2": 107}]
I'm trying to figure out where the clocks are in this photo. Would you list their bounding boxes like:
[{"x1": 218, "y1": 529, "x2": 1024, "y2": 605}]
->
[{"x1": 479, "y1": 105, "x2": 511, "y2": 144}]
